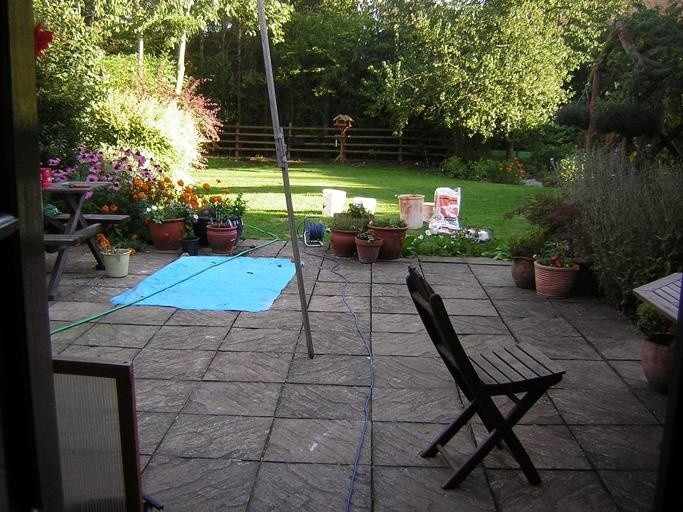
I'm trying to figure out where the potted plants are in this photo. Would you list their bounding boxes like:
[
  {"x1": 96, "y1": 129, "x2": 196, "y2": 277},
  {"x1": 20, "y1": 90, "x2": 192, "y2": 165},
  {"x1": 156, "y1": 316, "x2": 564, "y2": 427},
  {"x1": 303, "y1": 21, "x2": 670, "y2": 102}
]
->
[
  {"x1": 632, "y1": 301, "x2": 676, "y2": 395},
  {"x1": 503, "y1": 228, "x2": 548, "y2": 289},
  {"x1": 329, "y1": 202, "x2": 409, "y2": 265}
]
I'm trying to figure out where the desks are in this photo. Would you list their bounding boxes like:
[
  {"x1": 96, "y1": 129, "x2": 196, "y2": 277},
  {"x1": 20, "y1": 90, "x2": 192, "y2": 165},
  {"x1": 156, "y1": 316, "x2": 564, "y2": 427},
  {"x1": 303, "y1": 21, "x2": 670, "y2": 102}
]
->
[{"x1": 42, "y1": 182, "x2": 111, "y2": 301}]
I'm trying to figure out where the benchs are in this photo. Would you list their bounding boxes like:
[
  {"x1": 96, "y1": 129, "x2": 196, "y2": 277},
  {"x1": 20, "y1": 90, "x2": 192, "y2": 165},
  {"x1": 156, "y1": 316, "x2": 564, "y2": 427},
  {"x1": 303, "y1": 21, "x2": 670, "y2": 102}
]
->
[
  {"x1": 42, "y1": 232, "x2": 80, "y2": 250},
  {"x1": 55, "y1": 212, "x2": 131, "y2": 225}
]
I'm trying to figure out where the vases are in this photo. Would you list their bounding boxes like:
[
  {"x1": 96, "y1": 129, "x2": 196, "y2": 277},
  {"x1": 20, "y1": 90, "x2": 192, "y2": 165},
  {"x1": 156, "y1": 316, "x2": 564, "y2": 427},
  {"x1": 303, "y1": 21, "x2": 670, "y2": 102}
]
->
[
  {"x1": 100, "y1": 248, "x2": 131, "y2": 277},
  {"x1": 533, "y1": 260, "x2": 578, "y2": 298},
  {"x1": 145, "y1": 217, "x2": 243, "y2": 256}
]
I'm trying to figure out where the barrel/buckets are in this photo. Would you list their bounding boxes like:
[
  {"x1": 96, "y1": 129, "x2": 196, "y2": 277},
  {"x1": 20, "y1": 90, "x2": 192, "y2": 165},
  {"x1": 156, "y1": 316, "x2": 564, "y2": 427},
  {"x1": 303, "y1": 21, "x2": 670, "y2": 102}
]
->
[
  {"x1": 398, "y1": 194, "x2": 425, "y2": 230},
  {"x1": 352, "y1": 196, "x2": 376, "y2": 215},
  {"x1": 422, "y1": 201, "x2": 434, "y2": 223},
  {"x1": 322, "y1": 188, "x2": 347, "y2": 217}
]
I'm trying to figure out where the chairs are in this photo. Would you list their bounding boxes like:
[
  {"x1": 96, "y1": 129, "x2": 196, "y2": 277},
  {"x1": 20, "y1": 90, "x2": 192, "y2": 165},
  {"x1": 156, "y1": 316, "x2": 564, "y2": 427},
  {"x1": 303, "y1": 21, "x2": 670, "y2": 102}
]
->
[{"x1": 405, "y1": 264, "x2": 566, "y2": 490}]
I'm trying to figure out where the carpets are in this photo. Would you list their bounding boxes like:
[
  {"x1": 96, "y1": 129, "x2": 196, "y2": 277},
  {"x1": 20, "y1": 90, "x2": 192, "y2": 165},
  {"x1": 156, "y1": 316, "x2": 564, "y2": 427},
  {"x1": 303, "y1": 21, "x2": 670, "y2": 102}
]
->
[{"x1": 111, "y1": 255, "x2": 304, "y2": 313}]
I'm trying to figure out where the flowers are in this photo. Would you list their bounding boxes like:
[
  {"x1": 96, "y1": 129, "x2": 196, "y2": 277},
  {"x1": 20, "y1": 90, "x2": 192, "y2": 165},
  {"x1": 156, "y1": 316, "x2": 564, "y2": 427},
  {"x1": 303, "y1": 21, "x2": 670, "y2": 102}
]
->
[
  {"x1": 532, "y1": 238, "x2": 581, "y2": 272},
  {"x1": 131, "y1": 174, "x2": 247, "y2": 223},
  {"x1": 94, "y1": 232, "x2": 141, "y2": 256}
]
